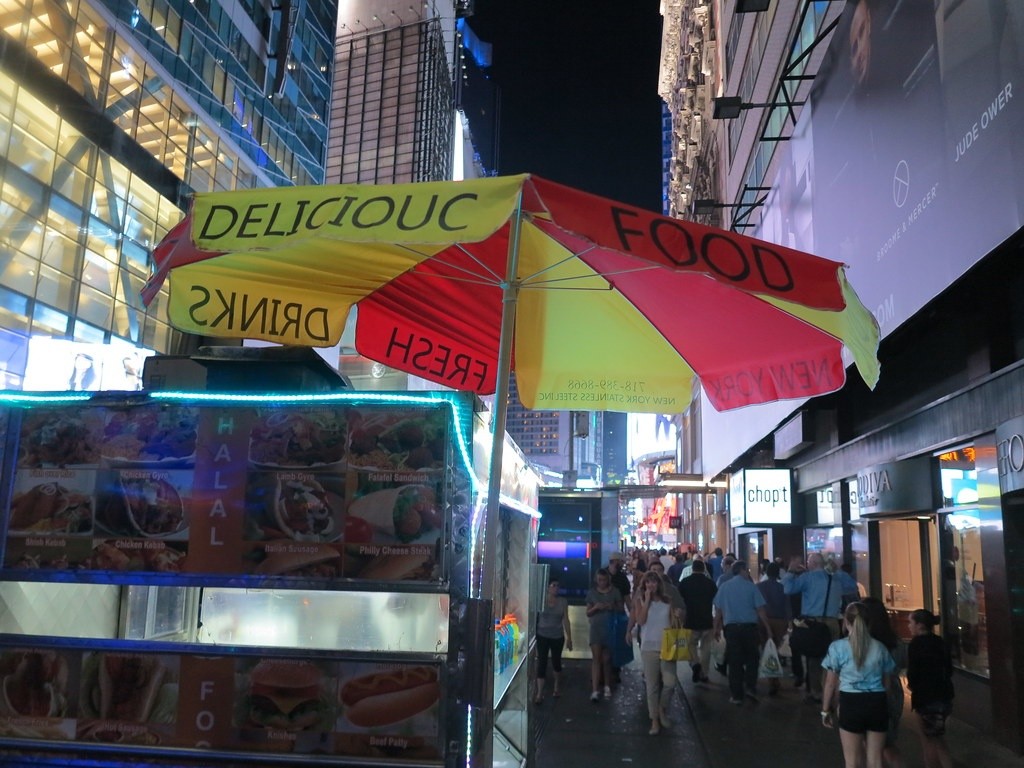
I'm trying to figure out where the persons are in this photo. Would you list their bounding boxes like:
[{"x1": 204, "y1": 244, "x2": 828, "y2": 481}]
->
[
  {"x1": 599, "y1": 550, "x2": 631, "y2": 682},
  {"x1": 711, "y1": 560, "x2": 775, "y2": 704},
  {"x1": 782, "y1": 556, "x2": 817, "y2": 693},
  {"x1": 631, "y1": 572, "x2": 684, "y2": 735},
  {"x1": 783, "y1": 553, "x2": 856, "y2": 700},
  {"x1": 860, "y1": 596, "x2": 906, "y2": 768},
  {"x1": 678, "y1": 560, "x2": 721, "y2": 681},
  {"x1": 531, "y1": 578, "x2": 575, "y2": 706},
  {"x1": 904, "y1": 607, "x2": 965, "y2": 768},
  {"x1": 832, "y1": 0, "x2": 905, "y2": 166},
  {"x1": 624, "y1": 542, "x2": 867, "y2": 671},
  {"x1": 754, "y1": 563, "x2": 793, "y2": 695},
  {"x1": 821, "y1": 600, "x2": 897, "y2": 767},
  {"x1": 583, "y1": 566, "x2": 626, "y2": 702},
  {"x1": 66, "y1": 353, "x2": 95, "y2": 392}
]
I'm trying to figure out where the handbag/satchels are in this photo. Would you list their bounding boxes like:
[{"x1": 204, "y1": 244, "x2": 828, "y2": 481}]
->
[
  {"x1": 790, "y1": 613, "x2": 832, "y2": 657},
  {"x1": 757, "y1": 637, "x2": 784, "y2": 679},
  {"x1": 659, "y1": 612, "x2": 694, "y2": 662}
]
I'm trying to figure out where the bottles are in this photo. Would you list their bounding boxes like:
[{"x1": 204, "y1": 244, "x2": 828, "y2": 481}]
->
[{"x1": 495, "y1": 613, "x2": 519, "y2": 676}]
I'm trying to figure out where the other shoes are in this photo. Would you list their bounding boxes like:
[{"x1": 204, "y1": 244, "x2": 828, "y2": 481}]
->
[
  {"x1": 553, "y1": 688, "x2": 561, "y2": 698},
  {"x1": 714, "y1": 661, "x2": 727, "y2": 675},
  {"x1": 649, "y1": 722, "x2": 659, "y2": 736},
  {"x1": 794, "y1": 675, "x2": 804, "y2": 687},
  {"x1": 660, "y1": 715, "x2": 671, "y2": 729},
  {"x1": 590, "y1": 691, "x2": 599, "y2": 702},
  {"x1": 747, "y1": 690, "x2": 761, "y2": 705},
  {"x1": 768, "y1": 680, "x2": 782, "y2": 696},
  {"x1": 729, "y1": 694, "x2": 744, "y2": 705},
  {"x1": 535, "y1": 695, "x2": 544, "y2": 705},
  {"x1": 604, "y1": 686, "x2": 611, "y2": 700},
  {"x1": 692, "y1": 663, "x2": 710, "y2": 683}
]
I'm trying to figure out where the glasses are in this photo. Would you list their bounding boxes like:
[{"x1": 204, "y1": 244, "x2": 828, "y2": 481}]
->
[{"x1": 547, "y1": 584, "x2": 561, "y2": 588}]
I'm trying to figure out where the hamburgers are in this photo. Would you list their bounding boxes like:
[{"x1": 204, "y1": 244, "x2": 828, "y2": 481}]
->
[{"x1": 247, "y1": 658, "x2": 324, "y2": 734}]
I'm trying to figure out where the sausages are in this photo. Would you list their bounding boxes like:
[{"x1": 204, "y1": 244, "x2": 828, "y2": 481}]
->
[
  {"x1": 348, "y1": 484, "x2": 441, "y2": 536},
  {"x1": 341, "y1": 666, "x2": 441, "y2": 728}
]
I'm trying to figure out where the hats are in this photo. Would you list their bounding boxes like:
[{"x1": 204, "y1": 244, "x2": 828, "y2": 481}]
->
[{"x1": 609, "y1": 552, "x2": 622, "y2": 561}]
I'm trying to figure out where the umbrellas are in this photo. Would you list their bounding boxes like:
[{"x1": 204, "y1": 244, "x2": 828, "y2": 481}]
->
[{"x1": 137, "y1": 173, "x2": 882, "y2": 595}]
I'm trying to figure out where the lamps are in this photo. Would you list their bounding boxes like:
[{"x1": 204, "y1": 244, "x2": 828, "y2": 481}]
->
[
  {"x1": 692, "y1": 197, "x2": 765, "y2": 216},
  {"x1": 711, "y1": 96, "x2": 807, "y2": 121},
  {"x1": 336, "y1": 1, "x2": 469, "y2": 82},
  {"x1": 735, "y1": 1, "x2": 769, "y2": 13}
]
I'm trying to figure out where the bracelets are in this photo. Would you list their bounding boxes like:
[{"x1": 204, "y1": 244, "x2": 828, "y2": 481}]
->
[{"x1": 821, "y1": 710, "x2": 833, "y2": 716}]
